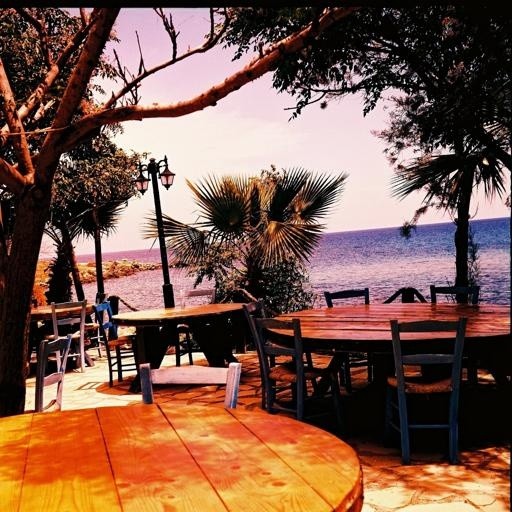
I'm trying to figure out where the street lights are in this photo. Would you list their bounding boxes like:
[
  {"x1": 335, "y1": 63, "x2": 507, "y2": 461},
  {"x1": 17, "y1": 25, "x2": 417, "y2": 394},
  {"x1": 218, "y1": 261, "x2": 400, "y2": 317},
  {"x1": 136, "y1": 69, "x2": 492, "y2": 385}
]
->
[{"x1": 138, "y1": 157, "x2": 176, "y2": 308}]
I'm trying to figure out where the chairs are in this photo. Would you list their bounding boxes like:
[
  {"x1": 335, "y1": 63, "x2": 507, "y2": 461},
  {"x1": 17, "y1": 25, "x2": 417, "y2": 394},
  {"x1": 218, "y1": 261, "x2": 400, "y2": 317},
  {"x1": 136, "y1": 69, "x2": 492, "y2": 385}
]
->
[
  {"x1": 92, "y1": 299, "x2": 263, "y2": 390},
  {"x1": 323, "y1": 284, "x2": 479, "y2": 384},
  {"x1": 19, "y1": 288, "x2": 101, "y2": 372},
  {"x1": 25, "y1": 332, "x2": 72, "y2": 413}
]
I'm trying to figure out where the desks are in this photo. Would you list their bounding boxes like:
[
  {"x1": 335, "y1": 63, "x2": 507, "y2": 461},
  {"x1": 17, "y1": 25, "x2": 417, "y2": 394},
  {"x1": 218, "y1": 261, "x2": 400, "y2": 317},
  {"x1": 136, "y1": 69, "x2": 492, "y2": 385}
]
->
[
  {"x1": 267, "y1": 303, "x2": 510, "y2": 439},
  {"x1": 1, "y1": 401, "x2": 367, "y2": 510}
]
